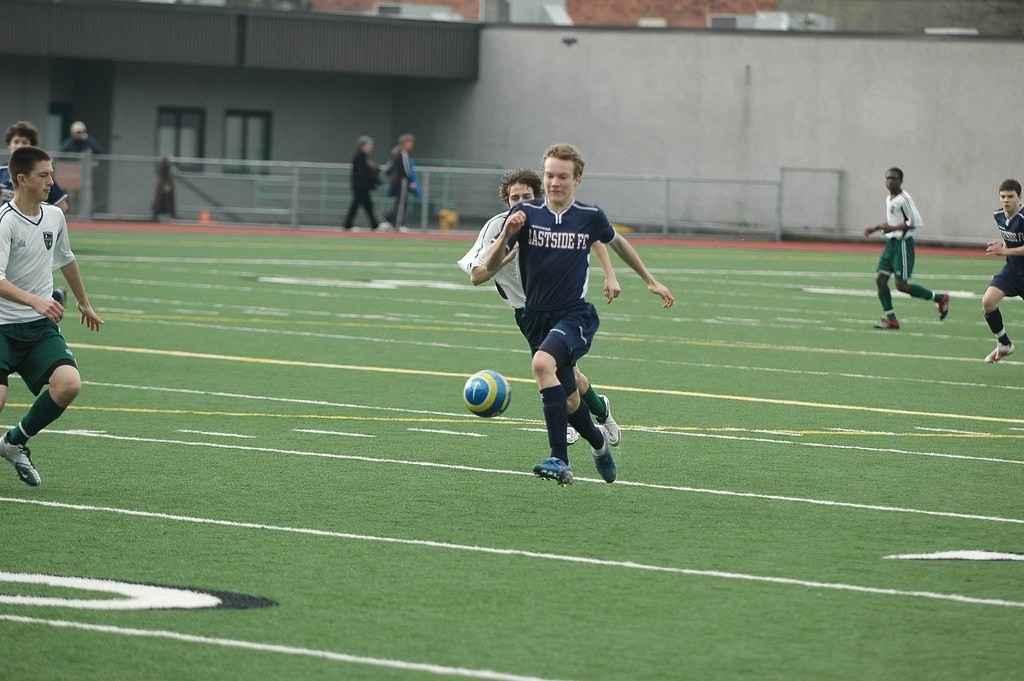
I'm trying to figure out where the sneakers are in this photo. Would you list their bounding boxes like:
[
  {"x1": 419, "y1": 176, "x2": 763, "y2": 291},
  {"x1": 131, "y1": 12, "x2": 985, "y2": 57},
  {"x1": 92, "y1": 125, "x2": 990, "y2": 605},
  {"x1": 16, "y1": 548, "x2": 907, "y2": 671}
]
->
[
  {"x1": 532, "y1": 457, "x2": 573, "y2": 488},
  {"x1": 936, "y1": 293, "x2": 949, "y2": 321},
  {"x1": 984, "y1": 341, "x2": 1014, "y2": 362},
  {"x1": 590, "y1": 425, "x2": 616, "y2": 483},
  {"x1": 0, "y1": 430, "x2": 42, "y2": 486},
  {"x1": 873, "y1": 319, "x2": 900, "y2": 329},
  {"x1": 566, "y1": 423, "x2": 580, "y2": 444},
  {"x1": 595, "y1": 395, "x2": 621, "y2": 447}
]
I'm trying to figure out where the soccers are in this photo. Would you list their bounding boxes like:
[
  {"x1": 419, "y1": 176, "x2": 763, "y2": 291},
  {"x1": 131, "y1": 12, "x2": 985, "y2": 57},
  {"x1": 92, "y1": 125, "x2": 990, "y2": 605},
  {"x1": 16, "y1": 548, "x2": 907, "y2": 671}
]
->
[{"x1": 462, "y1": 369, "x2": 513, "y2": 419}]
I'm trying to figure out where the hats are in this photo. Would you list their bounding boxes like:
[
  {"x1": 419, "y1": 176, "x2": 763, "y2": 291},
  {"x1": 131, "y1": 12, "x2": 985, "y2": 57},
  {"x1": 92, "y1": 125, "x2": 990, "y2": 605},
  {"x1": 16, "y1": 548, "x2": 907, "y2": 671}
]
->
[
  {"x1": 398, "y1": 132, "x2": 416, "y2": 143},
  {"x1": 69, "y1": 123, "x2": 87, "y2": 133},
  {"x1": 356, "y1": 134, "x2": 372, "y2": 144}
]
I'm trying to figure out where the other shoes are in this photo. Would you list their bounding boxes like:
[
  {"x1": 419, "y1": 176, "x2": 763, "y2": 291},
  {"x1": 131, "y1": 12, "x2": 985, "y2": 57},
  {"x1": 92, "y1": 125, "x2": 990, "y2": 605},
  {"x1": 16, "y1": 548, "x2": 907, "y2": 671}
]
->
[{"x1": 52, "y1": 287, "x2": 66, "y2": 320}]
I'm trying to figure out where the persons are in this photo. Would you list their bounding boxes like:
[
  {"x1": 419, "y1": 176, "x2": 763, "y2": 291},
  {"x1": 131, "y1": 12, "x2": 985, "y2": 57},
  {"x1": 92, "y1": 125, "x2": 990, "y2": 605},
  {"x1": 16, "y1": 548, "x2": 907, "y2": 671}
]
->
[
  {"x1": 342, "y1": 136, "x2": 379, "y2": 229},
  {"x1": 57, "y1": 122, "x2": 105, "y2": 212},
  {"x1": 862, "y1": 168, "x2": 950, "y2": 329},
  {"x1": 388, "y1": 134, "x2": 416, "y2": 233},
  {"x1": 0, "y1": 121, "x2": 68, "y2": 213},
  {"x1": 0, "y1": 146, "x2": 104, "y2": 487},
  {"x1": 488, "y1": 144, "x2": 675, "y2": 486},
  {"x1": 981, "y1": 179, "x2": 1024, "y2": 363},
  {"x1": 457, "y1": 167, "x2": 622, "y2": 447},
  {"x1": 152, "y1": 159, "x2": 176, "y2": 223}
]
